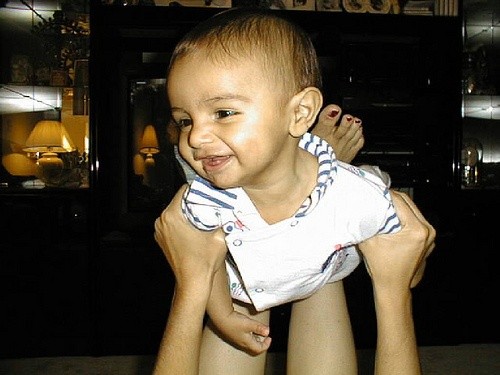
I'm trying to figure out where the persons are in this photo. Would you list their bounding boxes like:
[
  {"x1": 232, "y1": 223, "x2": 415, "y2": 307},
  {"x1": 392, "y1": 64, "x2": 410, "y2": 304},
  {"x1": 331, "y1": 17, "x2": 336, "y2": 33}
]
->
[
  {"x1": 152, "y1": 105, "x2": 436, "y2": 375},
  {"x1": 165, "y1": 9, "x2": 432, "y2": 356}
]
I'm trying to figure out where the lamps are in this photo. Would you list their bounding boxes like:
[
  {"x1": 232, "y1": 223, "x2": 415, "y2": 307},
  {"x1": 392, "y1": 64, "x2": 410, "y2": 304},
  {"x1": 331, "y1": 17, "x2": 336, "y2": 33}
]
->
[
  {"x1": 141, "y1": 125, "x2": 158, "y2": 162},
  {"x1": 23, "y1": 120, "x2": 75, "y2": 184}
]
